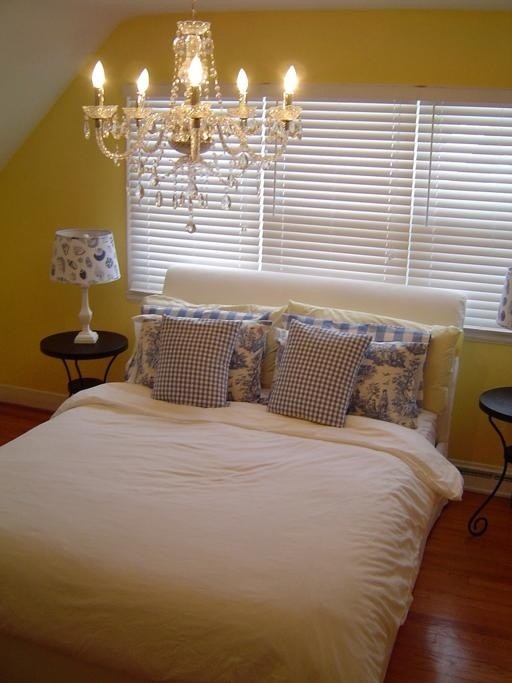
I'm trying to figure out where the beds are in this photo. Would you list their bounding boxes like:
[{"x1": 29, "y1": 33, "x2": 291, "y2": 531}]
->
[{"x1": 1, "y1": 265, "x2": 465, "y2": 682}]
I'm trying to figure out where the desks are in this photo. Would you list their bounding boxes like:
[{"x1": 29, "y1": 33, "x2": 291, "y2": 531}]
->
[
  {"x1": 467, "y1": 385, "x2": 512, "y2": 537},
  {"x1": 40, "y1": 330, "x2": 130, "y2": 398}
]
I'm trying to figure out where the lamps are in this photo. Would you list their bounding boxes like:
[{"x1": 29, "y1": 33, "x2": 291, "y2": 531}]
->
[
  {"x1": 498, "y1": 266, "x2": 512, "y2": 334},
  {"x1": 49, "y1": 227, "x2": 122, "y2": 346},
  {"x1": 79, "y1": 1, "x2": 306, "y2": 235}
]
